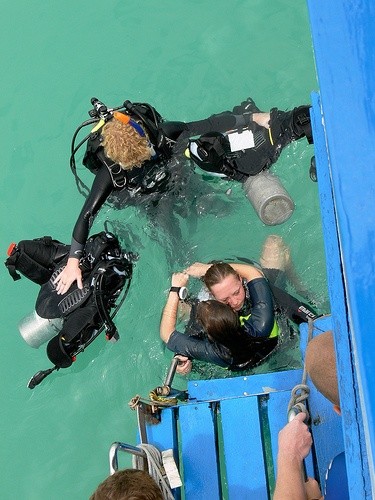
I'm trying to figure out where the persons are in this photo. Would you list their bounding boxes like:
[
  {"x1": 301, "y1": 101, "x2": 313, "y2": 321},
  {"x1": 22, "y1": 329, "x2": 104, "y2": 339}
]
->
[
  {"x1": 54, "y1": 98, "x2": 270, "y2": 295},
  {"x1": 160, "y1": 234, "x2": 319, "y2": 375},
  {"x1": 88, "y1": 411, "x2": 325, "y2": 500}
]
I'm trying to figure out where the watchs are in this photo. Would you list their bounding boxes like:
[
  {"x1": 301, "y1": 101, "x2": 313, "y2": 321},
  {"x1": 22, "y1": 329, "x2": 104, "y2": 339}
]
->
[{"x1": 168, "y1": 286, "x2": 189, "y2": 300}]
